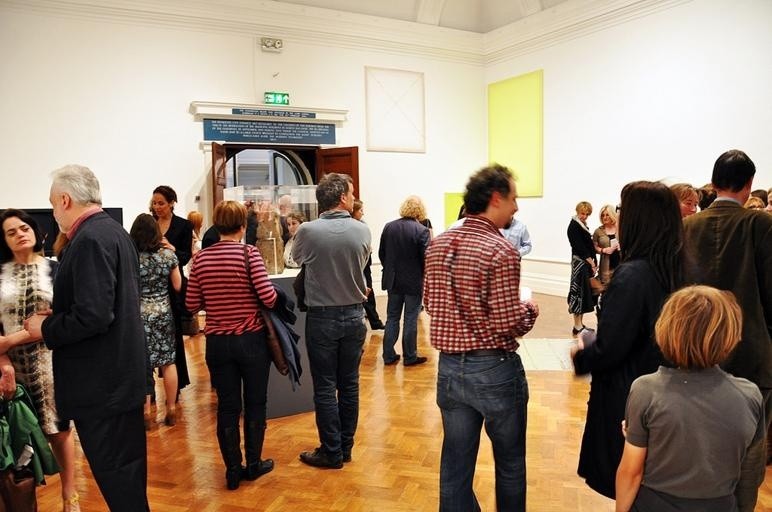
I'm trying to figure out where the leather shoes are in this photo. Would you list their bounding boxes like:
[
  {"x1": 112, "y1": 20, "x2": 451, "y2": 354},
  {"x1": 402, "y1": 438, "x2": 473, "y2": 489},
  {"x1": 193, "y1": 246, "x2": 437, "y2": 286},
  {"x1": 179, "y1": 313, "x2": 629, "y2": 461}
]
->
[
  {"x1": 372, "y1": 323, "x2": 385, "y2": 330},
  {"x1": 226, "y1": 464, "x2": 244, "y2": 489},
  {"x1": 299, "y1": 445, "x2": 351, "y2": 469},
  {"x1": 244, "y1": 459, "x2": 273, "y2": 481}
]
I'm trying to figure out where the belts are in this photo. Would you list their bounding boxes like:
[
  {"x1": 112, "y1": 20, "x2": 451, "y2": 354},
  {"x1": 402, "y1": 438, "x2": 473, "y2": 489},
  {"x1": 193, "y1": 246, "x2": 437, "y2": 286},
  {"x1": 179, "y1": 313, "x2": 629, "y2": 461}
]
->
[{"x1": 442, "y1": 349, "x2": 506, "y2": 357}]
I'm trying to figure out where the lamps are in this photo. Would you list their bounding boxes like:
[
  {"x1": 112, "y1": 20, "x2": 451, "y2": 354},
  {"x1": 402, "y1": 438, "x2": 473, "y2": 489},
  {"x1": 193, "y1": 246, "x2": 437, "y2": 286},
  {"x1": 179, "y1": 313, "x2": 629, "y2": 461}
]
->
[{"x1": 261, "y1": 37, "x2": 283, "y2": 52}]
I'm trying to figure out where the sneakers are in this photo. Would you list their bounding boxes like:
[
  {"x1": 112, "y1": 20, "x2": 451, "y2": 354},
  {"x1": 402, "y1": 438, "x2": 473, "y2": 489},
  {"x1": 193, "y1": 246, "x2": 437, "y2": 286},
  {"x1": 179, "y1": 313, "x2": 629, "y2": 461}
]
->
[
  {"x1": 405, "y1": 358, "x2": 427, "y2": 365},
  {"x1": 385, "y1": 355, "x2": 400, "y2": 365}
]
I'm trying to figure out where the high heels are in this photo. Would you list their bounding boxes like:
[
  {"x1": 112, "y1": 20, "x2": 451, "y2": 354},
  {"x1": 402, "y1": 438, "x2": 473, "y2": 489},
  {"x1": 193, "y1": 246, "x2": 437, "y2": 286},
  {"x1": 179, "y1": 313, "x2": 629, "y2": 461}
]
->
[
  {"x1": 261, "y1": 308, "x2": 290, "y2": 376},
  {"x1": 145, "y1": 413, "x2": 152, "y2": 431},
  {"x1": 165, "y1": 412, "x2": 176, "y2": 426}
]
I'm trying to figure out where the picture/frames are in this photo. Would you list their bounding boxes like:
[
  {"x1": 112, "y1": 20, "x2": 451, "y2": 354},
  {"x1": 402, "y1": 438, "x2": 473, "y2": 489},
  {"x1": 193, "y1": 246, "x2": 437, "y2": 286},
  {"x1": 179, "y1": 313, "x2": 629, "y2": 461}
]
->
[
  {"x1": 364, "y1": 66, "x2": 428, "y2": 155},
  {"x1": 487, "y1": 69, "x2": 544, "y2": 197}
]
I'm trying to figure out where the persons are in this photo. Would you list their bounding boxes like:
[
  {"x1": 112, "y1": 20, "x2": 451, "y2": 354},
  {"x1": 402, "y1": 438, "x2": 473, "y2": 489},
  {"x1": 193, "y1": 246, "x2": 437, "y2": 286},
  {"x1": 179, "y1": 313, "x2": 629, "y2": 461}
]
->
[
  {"x1": 278, "y1": 171, "x2": 387, "y2": 468},
  {"x1": 378, "y1": 197, "x2": 433, "y2": 365},
  {"x1": 23, "y1": 164, "x2": 151, "y2": 512},
  {"x1": 186, "y1": 195, "x2": 305, "y2": 269},
  {"x1": 185, "y1": 201, "x2": 277, "y2": 490},
  {"x1": 131, "y1": 186, "x2": 193, "y2": 428},
  {"x1": 567, "y1": 149, "x2": 772, "y2": 512},
  {"x1": 425, "y1": 164, "x2": 539, "y2": 512},
  {"x1": 1, "y1": 208, "x2": 81, "y2": 512}
]
